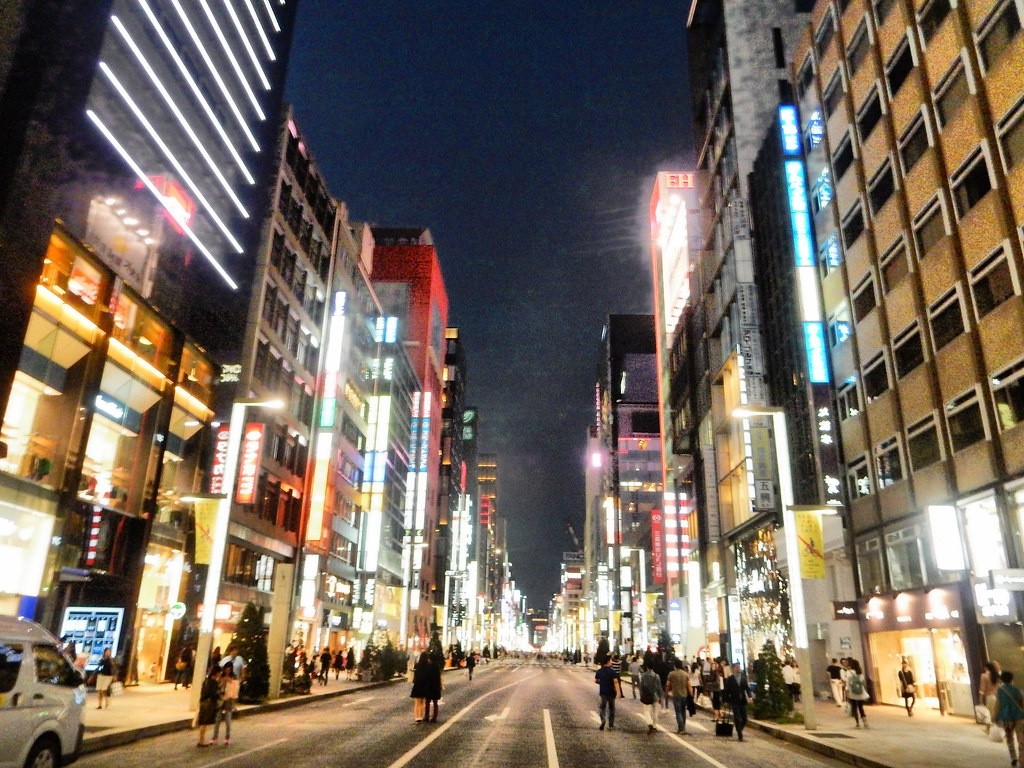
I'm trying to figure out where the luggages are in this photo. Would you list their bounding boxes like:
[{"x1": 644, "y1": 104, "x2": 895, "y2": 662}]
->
[{"x1": 715, "y1": 704, "x2": 733, "y2": 737}]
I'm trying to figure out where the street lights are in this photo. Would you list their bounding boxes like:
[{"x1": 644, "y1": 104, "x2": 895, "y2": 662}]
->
[
  {"x1": 190, "y1": 395, "x2": 286, "y2": 712},
  {"x1": 732, "y1": 405, "x2": 819, "y2": 730}
]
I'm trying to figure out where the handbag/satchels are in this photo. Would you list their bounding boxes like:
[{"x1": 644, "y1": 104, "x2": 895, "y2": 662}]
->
[
  {"x1": 904, "y1": 684, "x2": 916, "y2": 695},
  {"x1": 109, "y1": 681, "x2": 124, "y2": 697},
  {"x1": 224, "y1": 679, "x2": 240, "y2": 701},
  {"x1": 974, "y1": 705, "x2": 992, "y2": 727},
  {"x1": 174, "y1": 659, "x2": 186, "y2": 671}
]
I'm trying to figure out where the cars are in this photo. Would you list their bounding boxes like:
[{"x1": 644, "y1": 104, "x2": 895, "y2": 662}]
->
[{"x1": 0, "y1": 615, "x2": 87, "y2": 767}]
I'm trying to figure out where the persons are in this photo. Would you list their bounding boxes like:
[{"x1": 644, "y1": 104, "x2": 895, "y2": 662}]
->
[
  {"x1": 410, "y1": 652, "x2": 441, "y2": 723},
  {"x1": 628, "y1": 650, "x2": 751, "y2": 741},
  {"x1": 825, "y1": 657, "x2": 870, "y2": 729},
  {"x1": 174, "y1": 643, "x2": 197, "y2": 690},
  {"x1": 319, "y1": 648, "x2": 332, "y2": 685},
  {"x1": 594, "y1": 655, "x2": 619, "y2": 732},
  {"x1": 333, "y1": 650, "x2": 343, "y2": 680},
  {"x1": 898, "y1": 660, "x2": 916, "y2": 716},
  {"x1": 611, "y1": 655, "x2": 625, "y2": 698},
  {"x1": 92, "y1": 647, "x2": 117, "y2": 710},
  {"x1": 780, "y1": 659, "x2": 802, "y2": 701},
  {"x1": 467, "y1": 654, "x2": 475, "y2": 680},
  {"x1": 345, "y1": 648, "x2": 354, "y2": 681},
  {"x1": 67, "y1": 643, "x2": 77, "y2": 660},
  {"x1": 978, "y1": 660, "x2": 1024, "y2": 765},
  {"x1": 207, "y1": 646, "x2": 247, "y2": 683},
  {"x1": 196, "y1": 661, "x2": 237, "y2": 747}
]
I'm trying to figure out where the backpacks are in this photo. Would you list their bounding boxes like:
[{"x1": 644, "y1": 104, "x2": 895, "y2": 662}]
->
[
  {"x1": 642, "y1": 674, "x2": 659, "y2": 697},
  {"x1": 846, "y1": 670, "x2": 864, "y2": 697}
]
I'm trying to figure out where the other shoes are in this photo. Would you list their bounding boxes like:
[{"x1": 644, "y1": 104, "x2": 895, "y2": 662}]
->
[
  {"x1": 174, "y1": 686, "x2": 180, "y2": 691},
  {"x1": 1010, "y1": 759, "x2": 1019, "y2": 767},
  {"x1": 609, "y1": 725, "x2": 614, "y2": 731},
  {"x1": 679, "y1": 729, "x2": 686, "y2": 736},
  {"x1": 739, "y1": 735, "x2": 745, "y2": 741},
  {"x1": 185, "y1": 684, "x2": 191, "y2": 689},
  {"x1": 414, "y1": 717, "x2": 437, "y2": 723},
  {"x1": 209, "y1": 738, "x2": 217, "y2": 746},
  {"x1": 599, "y1": 720, "x2": 607, "y2": 731},
  {"x1": 907, "y1": 707, "x2": 913, "y2": 717},
  {"x1": 96, "y1": 704, "x2": 108, "y2": 710},
  {"x1": 224, "y1": 739, "x2": 230, "y2": 746},
  {"x1": 861, "y1": 717, "x2": 870, "y2": 728},
  {"x1": 647, "y1": 725, "x2": 658, "y2": 734},
  {"x1": 855, "y1": 723, "x2": 863, "y2": 729},
  {"x1": 197, "y1": 742, "x2": 209, "y2": 748}
]
